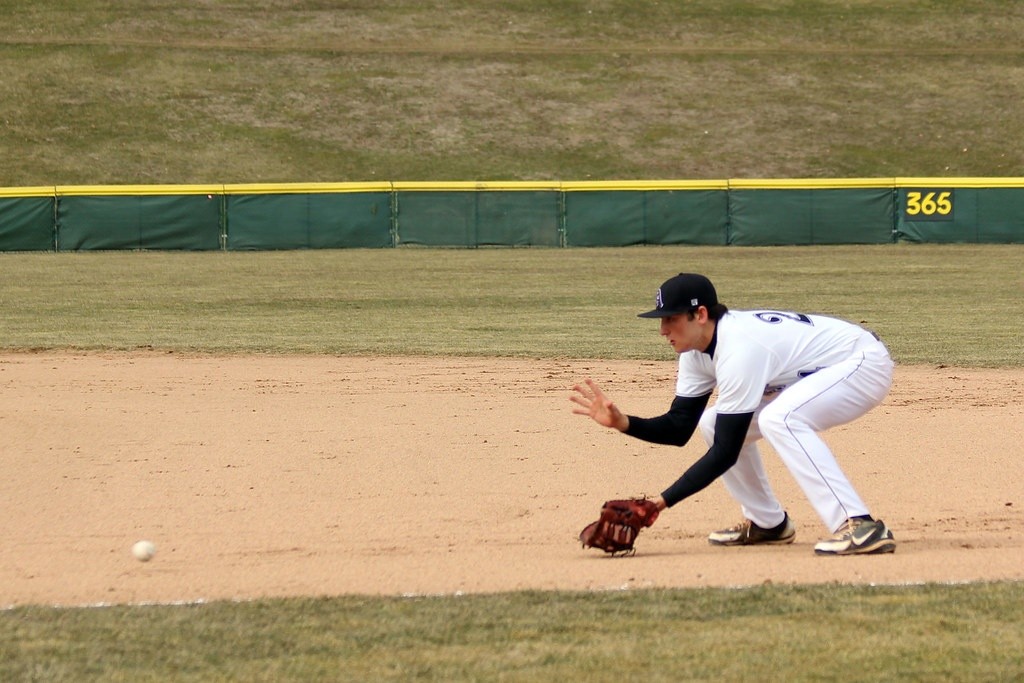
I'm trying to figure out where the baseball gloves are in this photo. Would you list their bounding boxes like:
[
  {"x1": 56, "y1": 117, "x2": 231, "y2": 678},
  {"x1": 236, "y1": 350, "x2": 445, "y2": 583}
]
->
[{"x1": 573, "y1": 495, "x2": 660, "y2": 560}]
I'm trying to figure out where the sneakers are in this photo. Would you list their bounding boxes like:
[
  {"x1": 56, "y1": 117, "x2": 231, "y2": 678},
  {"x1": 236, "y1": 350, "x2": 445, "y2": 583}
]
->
[
  {"x1": 815, "y1": 519, "x2": 896, "y2": 555},
  {"x1": 708, "y1": 512, "x2": 796, "y2": 547}
]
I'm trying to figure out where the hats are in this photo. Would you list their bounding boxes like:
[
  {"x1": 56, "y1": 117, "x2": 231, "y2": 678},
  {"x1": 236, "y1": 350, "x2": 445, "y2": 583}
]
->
[{"x1": 638, "y1": 272, "x2": 720, "y2": 318}]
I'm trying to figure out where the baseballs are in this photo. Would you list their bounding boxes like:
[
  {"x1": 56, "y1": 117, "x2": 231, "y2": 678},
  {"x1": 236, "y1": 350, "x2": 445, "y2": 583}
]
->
[{"x1": 133, "y1": 539, "x2": 157, "y2": 562}]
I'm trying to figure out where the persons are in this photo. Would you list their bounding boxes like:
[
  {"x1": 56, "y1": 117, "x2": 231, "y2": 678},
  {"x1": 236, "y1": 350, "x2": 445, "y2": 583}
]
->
[{"x1": 569, "y1": 271, "x2": 899, "y2": 559}]
